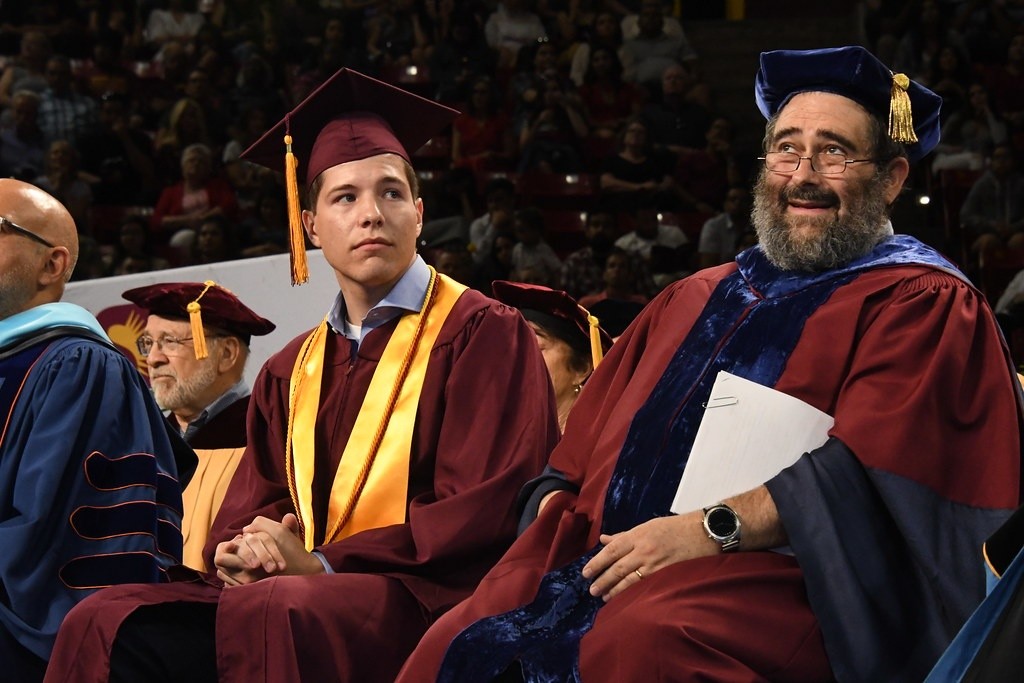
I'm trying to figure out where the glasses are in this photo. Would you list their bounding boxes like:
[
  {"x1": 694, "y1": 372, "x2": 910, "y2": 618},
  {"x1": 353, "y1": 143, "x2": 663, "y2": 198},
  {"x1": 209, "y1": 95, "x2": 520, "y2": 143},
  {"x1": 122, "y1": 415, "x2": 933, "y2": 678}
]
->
[
  {"x1": 754, "y1": 147, "x2": 887, "y2": 180},
  {"x1": 46, "y1": 69, "x2": 71, "y2": 79}
]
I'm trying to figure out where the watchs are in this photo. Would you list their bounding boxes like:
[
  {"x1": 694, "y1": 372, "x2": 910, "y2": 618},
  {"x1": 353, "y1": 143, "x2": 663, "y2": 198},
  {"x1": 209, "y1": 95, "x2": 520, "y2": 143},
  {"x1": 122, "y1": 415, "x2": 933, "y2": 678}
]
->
[{"x1": 701, "y1": 501, "x2": 742, "y2": 555}]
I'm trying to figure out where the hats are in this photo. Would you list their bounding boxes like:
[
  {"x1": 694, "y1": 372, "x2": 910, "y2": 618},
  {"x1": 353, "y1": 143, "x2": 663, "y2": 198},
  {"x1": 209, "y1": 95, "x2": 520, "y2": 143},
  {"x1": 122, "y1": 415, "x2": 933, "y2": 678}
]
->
[
  {"x1": 236, "y1": 65, "x2": 469, "y2": 290},
  {"x1": 751, "y1": 47, "x2": 942, "y2": 167},
  {"x1": 490, "y1": 279, "x2": 617, "y2": 376},
  {"x1": 117, "y1": 280, "x2": 278, "y2": 357}
]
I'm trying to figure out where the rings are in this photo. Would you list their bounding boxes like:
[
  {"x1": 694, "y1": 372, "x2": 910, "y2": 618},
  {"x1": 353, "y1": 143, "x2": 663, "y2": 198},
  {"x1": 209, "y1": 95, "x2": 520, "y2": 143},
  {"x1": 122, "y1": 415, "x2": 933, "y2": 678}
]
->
[{"x1": 634, "y1": 570, "x2": 644, "y2": 580}]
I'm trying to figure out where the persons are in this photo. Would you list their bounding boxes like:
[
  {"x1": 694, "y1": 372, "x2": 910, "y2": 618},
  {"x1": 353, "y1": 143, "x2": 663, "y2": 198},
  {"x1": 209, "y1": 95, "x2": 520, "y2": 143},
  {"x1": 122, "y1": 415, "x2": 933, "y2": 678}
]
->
[
  {"x1": 122, "y1": 279, "x2": 279, "y2": 575},
  {"x1": 389, "y1": 43, "x2": 1022, "y2": 683},
  {"x1": 487, "y1": 277, "x2": 616, "y2": 446},
  {"x1": 40, "y1": 65, "x2": 563, "y2": 682},
  {"x1": 0, "y1": 178, "x2": 185, "y2": 683},
  {"x1": 0, "y1": 0, "x2": 1023, "y2": 375}
]
